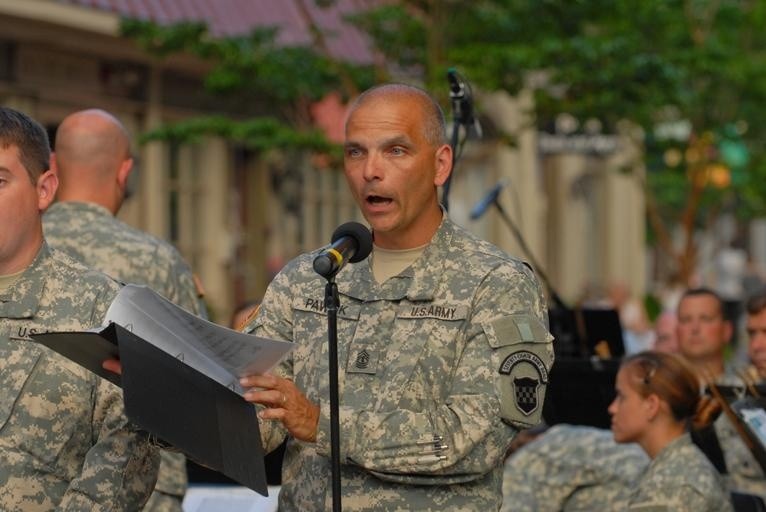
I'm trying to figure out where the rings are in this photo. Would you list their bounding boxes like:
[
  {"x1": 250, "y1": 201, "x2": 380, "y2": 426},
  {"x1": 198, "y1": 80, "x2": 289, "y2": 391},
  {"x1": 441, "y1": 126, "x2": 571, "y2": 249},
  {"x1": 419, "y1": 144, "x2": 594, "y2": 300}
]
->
[{"x1": 280, "y1": 392, "x2": 288, "y2": 408}]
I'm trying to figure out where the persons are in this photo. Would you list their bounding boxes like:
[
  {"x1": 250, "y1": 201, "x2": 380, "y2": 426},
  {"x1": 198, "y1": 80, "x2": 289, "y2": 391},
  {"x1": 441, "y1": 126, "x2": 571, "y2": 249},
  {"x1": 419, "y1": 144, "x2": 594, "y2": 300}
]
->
[
  {"x1": 104, "y1": 82, "x2": 556, "y2": 512},
  {"x1": 501, "y1": 420, "x2": 650, "y2": 512},
  {"x1": 0, "y1": 106, "x2": 160, "y2": 512},
  {"x1": 41, "y1": 108, "x2": 207, "y2": 512},
  {"x1": 603, "y1": 352, "x2": 735, "y2": 511},
  {"x1": 673, "y1": 288, "x2": 734, "y2": 376},
  {"x1": 745, "y1": 286, "x2": 766, "y2": 385}
]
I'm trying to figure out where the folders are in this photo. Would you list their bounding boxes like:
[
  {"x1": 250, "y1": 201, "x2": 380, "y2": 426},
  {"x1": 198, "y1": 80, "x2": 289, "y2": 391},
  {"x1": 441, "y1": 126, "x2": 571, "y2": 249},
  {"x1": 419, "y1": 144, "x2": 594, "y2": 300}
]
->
[{"x1": 29, "y1": 284, "x2": 296, "y2": 498}]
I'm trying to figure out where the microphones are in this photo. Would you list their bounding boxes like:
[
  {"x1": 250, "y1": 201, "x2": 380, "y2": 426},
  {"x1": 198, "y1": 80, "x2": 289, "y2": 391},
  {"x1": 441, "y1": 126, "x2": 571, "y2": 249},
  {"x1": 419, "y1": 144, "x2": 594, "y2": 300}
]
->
[
  {"x1": 469, "y1": 177, "x2": 509, "y2": 221},
  {"x1": 312, "y1": 221, "x2": 374, "y2": 278},
  {"x1": 447, "y1": 70, "x2": 484, "y2": 141}
]
